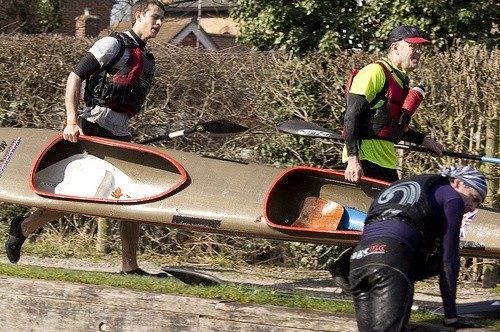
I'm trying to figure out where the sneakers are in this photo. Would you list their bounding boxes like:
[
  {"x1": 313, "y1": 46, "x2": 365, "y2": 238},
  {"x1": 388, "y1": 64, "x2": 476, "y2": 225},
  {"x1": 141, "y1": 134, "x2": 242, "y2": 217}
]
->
[
  {"x1": 119, "y1": 268, "x2": 168, "y2": 279},
  {"x1": 5, "y1": 215, "x2": 28, "y2": 264}
]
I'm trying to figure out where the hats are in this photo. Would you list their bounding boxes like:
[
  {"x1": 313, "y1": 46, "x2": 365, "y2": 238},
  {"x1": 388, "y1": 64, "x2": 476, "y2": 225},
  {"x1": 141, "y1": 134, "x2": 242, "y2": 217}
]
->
[{"x1": 387, "y1": 25, "x2": 432, "y2": 48}]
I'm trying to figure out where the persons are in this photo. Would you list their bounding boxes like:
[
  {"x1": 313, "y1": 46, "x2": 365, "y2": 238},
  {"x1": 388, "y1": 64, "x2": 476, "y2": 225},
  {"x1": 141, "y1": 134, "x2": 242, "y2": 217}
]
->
[
  {"x1": 327, "y1": 25, "x2": 444, "y2": 296},
  {"x1": 6, "y1": 0, "x2": 169, "y2": 278},
  {"x1": 349, "y1": 166, "x2": 488, "y2": 332}
]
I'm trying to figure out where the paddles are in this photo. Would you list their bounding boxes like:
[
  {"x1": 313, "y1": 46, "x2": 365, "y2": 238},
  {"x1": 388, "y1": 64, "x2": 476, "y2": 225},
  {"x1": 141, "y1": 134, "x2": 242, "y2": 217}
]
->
[
  {"x1": 277, "y1": 113, "x2": 500, "y2": 167},
  {"x1": 137, "y1": 114, "x2": 249, "y2": 145},
  {"x1": 159, "y1": 262, "x2": 500, "y2": 316}
]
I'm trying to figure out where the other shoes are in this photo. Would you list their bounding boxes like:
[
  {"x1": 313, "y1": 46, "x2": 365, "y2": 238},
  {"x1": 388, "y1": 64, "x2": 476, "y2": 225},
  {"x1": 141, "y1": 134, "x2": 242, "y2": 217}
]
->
[{"x1": 328, "y1": 258, "x2": 352, "y2": 295}]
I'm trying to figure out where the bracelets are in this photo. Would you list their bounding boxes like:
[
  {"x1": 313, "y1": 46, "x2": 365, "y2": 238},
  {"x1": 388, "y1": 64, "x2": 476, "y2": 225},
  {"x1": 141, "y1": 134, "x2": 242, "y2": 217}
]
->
[{"x1": 67, "y1": 121, "x2": 78, "y2": 125}]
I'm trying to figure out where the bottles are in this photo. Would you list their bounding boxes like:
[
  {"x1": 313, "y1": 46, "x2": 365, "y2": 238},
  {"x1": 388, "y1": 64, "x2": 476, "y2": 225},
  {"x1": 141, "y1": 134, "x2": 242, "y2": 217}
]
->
[{"x1": 402, "y1": 82, "x2": 426, "y2": 114}]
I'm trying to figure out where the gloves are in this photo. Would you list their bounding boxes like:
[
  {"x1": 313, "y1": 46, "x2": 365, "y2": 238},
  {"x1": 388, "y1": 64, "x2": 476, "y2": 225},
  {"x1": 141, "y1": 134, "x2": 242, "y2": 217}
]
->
[{"x1": 443, "y1": 316, "x2": 483, "y2": 328}]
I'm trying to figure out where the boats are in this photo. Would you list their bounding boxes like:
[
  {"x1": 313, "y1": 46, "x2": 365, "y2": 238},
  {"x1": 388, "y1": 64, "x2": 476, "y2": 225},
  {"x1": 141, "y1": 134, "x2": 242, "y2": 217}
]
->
[{"x1": 0, "y1": 127, "x2": 500, "y2": 259}]
